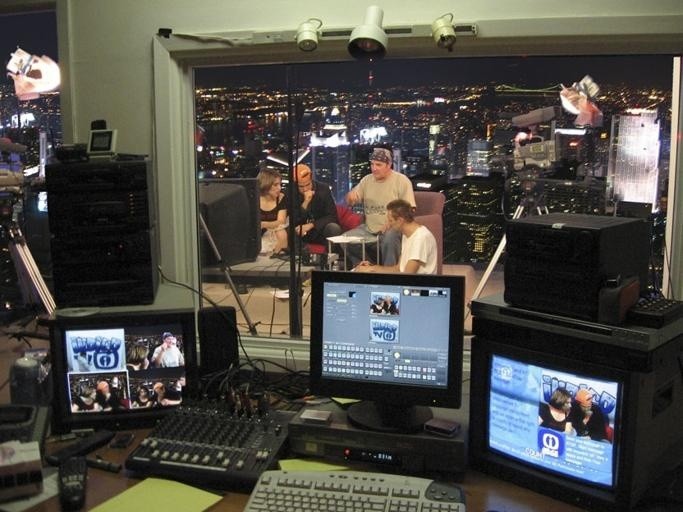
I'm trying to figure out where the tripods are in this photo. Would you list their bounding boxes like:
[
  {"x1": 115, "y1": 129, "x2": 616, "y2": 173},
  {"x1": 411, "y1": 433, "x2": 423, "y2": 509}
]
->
[
  {"x1": 0, "y1": 217, "x2": 57, "y2": 316},
  {"x1": 465, "y1": 206, "x2": 549, "y2": 320}
]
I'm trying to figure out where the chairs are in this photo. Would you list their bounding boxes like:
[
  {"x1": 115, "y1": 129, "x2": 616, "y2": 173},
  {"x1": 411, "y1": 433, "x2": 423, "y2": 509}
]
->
[
  {"x1": 307, "y1": 205, "x2": 361, "y2": 252},
  {"x1": 415, "y1": 191, "x2": 446, "y2": 273}
]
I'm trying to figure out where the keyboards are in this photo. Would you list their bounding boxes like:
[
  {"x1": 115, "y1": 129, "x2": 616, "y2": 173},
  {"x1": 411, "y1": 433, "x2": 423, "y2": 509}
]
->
[{"x1": 242, "y1": 470, "x2": 467, "y2": 512}]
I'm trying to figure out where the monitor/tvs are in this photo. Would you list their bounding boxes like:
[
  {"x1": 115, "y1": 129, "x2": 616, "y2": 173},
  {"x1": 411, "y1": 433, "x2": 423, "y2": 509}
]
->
[
  {"x1": 310, "y1": 271, "x2": 465, "y2": 433},
  {"x1": 469, "y1": 313, "x2": 682, "y2": 512},
  {"x1": 197, "y1": 178, "x2": 261, "y2": 268},
  {"x1": 49, "y1": 304, "x2": 237, "y2": 434}
]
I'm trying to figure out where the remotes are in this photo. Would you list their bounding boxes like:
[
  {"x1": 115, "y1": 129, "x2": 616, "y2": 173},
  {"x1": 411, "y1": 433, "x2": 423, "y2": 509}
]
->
[
  {"x1": 58, "y1": 456, "x2": 87, "y2": 510},
  {"x1": 46, "y1": 427, "x2": 115, "y2": 465}
]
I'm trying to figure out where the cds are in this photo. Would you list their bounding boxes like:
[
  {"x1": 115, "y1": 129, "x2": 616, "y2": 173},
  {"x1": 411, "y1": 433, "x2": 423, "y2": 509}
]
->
[{"x1": 54, "y1": 306, "x2": 99, "y2": 318}]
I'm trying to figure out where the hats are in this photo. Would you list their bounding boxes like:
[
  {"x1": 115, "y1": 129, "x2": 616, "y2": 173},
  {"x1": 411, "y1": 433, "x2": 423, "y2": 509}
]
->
[{"x1": 575, "y1": 389, "x2": 593, "y2": 408}]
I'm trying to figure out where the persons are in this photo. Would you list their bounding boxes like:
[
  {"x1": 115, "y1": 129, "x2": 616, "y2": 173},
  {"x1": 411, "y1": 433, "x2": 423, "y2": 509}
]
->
[
  {"x1": 286, "y1": 164, "x2": 352, "y2": 271},
  {"x1": 257, "y1": 169, "x2": 287, "y2": 236},
  {"x1": 566, "y1": 389, "x2": 604, "y2": 442},
  {"x1": 357, "y1": 199, "x2": 438, "y2": 275},
  {"x1": 370, "y1": 295, "x2": 397, "y2": 316},
  {"x1": 539, "y1": 387, "x2": 576, "y2": 434},
  {"x1": 72, "y1": 331, "x2": 185, "y2": 412},
  {"x1": 339, "y1": 147, "x2": 416, "y2": 266}
]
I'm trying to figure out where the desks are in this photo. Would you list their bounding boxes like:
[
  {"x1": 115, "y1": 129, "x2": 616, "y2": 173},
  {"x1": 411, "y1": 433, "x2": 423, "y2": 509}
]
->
[
  {"x1": 49, "y1": 283, "x2": 196, "y2": 344},
  {"x1": 327, "y1": 234, "x2": 381, "y2": 271}
]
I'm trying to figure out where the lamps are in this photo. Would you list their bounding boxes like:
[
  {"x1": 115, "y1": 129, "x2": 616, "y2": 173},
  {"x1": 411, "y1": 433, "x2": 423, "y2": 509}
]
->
[
  {"x1": 297, "y1": 8, "x2": 458, "y2": 60},
  {"x1": 7, "y1": 46, "x2": 60, "y2": 103},
  {"x1": 559, "y1": 75, "x2": 606, "y2": 129}
]
